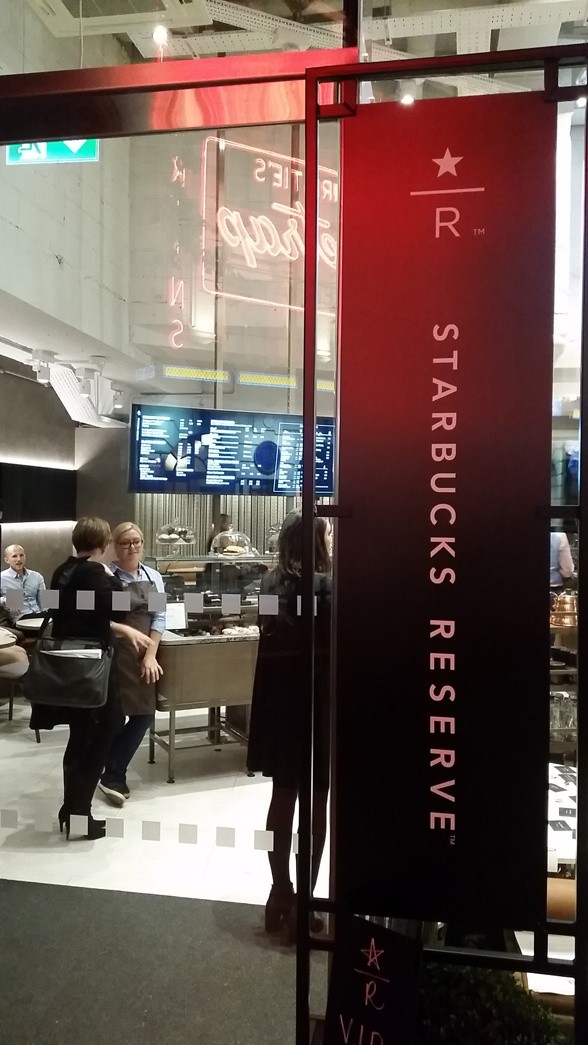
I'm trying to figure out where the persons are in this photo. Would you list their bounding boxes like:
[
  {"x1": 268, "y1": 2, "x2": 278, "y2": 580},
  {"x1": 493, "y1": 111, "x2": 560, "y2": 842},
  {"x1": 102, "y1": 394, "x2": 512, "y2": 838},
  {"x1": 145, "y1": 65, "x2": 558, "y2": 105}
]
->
[
  {"x1": 202, "y1": 513, "x2": 232, "y2": 583},
  {"x1": 550, "y1": 529, "x2": 575, "y2": 593},
  {"x1": 26, "y1": 517, "x2": 127, "y2": 841},
  {"x1": 0, "y1": 544, "x2": 49, "y2": 639},
  {"x1": 98, "y1": 520, "x2": 167, "y2": 807},
  {"x1": 241, "y1": 509, "x2": 333, "y2": 935}
]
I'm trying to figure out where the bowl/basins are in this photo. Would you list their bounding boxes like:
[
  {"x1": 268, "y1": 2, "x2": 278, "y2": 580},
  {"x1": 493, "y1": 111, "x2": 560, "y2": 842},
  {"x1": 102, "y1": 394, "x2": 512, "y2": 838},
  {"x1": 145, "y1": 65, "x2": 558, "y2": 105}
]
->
[{"x1": 550, "y1": 661, "x2": 565, "y2": 669}]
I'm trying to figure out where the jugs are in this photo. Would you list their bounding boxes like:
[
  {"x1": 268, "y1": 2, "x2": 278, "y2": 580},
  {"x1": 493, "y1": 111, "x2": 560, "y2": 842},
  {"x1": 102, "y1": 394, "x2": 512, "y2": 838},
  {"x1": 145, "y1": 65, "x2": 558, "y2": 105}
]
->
[{"x1": 549, "y1": 588, "x2": 578, "y2": 626}]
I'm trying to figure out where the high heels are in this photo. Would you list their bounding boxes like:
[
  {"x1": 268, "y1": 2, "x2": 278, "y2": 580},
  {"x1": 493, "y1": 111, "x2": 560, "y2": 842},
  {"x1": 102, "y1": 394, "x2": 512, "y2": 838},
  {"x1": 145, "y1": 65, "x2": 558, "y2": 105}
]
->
[
  {"x1": 263, "y1": 883, "x2": 326, "y2": 935},
  {"x1": 58, "y1": 803, "x2": 106, "y2": 832},
  {"x1": 66, "y1": 804, "x2": 106, "y2": 840}
]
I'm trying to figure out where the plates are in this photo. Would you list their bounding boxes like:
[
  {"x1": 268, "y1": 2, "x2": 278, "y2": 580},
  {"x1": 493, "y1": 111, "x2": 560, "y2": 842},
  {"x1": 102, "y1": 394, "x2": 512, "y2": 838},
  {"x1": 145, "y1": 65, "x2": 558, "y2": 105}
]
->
[{"x1": 223, "y1": 553, "x2": 240, "y2": 556}]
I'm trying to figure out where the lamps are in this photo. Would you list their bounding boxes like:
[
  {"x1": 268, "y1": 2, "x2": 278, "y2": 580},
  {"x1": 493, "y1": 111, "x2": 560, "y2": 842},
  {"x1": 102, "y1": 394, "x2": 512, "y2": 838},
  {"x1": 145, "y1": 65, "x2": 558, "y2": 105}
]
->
[{"x1": 369, "y1": 82, "x2": 401, "y2": 103}]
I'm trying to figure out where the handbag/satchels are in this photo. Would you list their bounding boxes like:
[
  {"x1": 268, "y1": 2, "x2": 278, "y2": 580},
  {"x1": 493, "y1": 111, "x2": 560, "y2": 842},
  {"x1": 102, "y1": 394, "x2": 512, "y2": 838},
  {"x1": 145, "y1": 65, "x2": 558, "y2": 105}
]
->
[{"x1": 24, "y1": 636, "x2": 113, "y2": 709}]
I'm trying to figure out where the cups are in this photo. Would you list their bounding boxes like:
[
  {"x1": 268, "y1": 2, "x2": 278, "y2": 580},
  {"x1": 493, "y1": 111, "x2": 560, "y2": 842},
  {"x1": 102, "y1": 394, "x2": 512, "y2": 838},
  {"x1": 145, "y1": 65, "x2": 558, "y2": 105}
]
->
[{"x1": 549, "y1": 691, "x2": 577, "y2": 742}]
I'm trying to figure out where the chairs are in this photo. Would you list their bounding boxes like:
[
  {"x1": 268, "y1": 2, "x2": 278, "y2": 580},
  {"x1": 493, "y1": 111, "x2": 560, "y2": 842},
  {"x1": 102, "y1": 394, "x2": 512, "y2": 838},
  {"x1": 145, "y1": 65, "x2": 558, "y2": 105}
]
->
[{"x1": 8, "y1": 608, "x2": 57, "y2": 743}]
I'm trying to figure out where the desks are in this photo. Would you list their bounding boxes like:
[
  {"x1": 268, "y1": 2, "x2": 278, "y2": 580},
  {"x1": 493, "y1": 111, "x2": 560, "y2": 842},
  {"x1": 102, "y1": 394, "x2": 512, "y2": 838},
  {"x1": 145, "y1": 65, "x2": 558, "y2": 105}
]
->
[{"x1": 144, "y1": 635, "x2": 265, "y2": 785}]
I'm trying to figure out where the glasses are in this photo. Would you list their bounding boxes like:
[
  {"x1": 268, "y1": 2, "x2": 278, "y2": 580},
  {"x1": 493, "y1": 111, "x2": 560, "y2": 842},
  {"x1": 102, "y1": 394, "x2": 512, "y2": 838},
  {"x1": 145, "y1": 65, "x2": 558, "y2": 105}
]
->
[{"x1": 117, "y1": 540, "x2": 143, "y2": 549}]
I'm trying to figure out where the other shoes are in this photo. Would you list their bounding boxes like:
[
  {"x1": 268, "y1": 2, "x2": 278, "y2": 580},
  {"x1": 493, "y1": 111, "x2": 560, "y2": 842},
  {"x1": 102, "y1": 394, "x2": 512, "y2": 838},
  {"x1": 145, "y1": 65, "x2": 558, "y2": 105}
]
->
[
  {"x1": 97, "y1": 772, "x2": 125, "y2": 806},
  {"x1": 123, "y1": 784, "x2": 129, "y2": 798}
]
